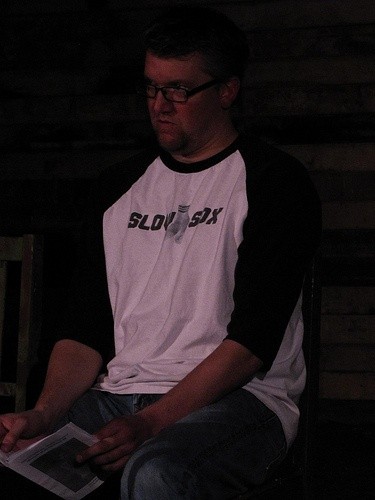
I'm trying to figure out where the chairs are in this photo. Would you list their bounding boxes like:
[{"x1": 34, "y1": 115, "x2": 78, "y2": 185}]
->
[{"x1": 0, "y1": 235, "x2": 44, "y2": 416}]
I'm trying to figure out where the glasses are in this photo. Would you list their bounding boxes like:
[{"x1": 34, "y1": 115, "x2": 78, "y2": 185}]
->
[{"x1": 143, "y1": 77, "x2": 227, "y2": 105}]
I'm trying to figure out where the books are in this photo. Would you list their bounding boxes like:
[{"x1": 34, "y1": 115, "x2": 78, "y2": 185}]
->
[{"x1": 0, "y1": 421, "x2": 105, "y2": 500}]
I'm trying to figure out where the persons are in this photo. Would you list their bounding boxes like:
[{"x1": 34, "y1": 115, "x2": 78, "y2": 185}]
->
[{"x1": 0, "y1": 4, "x2": 322, "y2": 500}]
